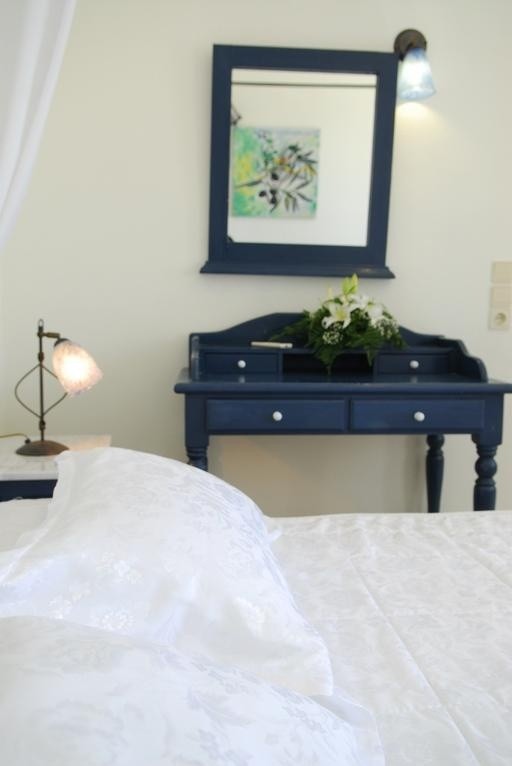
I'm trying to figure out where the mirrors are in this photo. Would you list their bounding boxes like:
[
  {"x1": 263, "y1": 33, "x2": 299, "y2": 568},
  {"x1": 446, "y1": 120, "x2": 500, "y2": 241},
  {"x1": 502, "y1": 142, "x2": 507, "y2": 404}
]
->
[{"x1": 200, "y1": 44, "x2": 399, "y2": 278}]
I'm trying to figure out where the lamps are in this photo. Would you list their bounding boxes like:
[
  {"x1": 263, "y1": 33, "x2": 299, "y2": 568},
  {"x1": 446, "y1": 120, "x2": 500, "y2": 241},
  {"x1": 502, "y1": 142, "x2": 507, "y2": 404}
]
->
[
  {"x1": 14, "y1": 318, "x2": 104, "y2": 455},
  {"x1": 394, "y1": 29, "x2": 437, "y2": 101}
]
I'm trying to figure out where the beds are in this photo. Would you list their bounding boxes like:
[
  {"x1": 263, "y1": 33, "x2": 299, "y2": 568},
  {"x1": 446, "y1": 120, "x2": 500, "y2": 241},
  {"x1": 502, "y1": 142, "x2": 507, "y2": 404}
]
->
[{"x1": 1, "y1": 448, "x2": 512, "y2": 766}]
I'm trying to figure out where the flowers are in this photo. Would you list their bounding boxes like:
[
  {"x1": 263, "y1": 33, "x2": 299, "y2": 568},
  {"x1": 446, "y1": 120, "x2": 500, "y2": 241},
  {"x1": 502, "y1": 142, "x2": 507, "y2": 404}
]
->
[{"x1": 266, "y1": 273, "x2": 409, "y2": 375}]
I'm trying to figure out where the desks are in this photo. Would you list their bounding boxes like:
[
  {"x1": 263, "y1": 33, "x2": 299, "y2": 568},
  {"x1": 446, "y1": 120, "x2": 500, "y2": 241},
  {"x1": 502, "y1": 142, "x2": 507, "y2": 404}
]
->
[{"x1": 175, "y1": 311, "x2": 512, "y2": 513}]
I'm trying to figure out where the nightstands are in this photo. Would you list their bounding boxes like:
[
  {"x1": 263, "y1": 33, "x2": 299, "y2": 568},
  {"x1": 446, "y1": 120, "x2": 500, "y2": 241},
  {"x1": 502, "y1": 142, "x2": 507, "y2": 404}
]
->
[{"x1": 0, "y1": 435, "x2": 111, "y2": 501}]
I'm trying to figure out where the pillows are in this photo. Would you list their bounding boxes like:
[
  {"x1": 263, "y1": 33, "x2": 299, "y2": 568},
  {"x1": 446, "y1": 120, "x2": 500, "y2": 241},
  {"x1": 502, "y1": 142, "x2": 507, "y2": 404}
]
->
[
  {"x1": 0, "y1": 449, "x2": 332, "y2": 694},
  {"x1": 1, "y1": 614, "x2": 382, "y2": 764}
]
